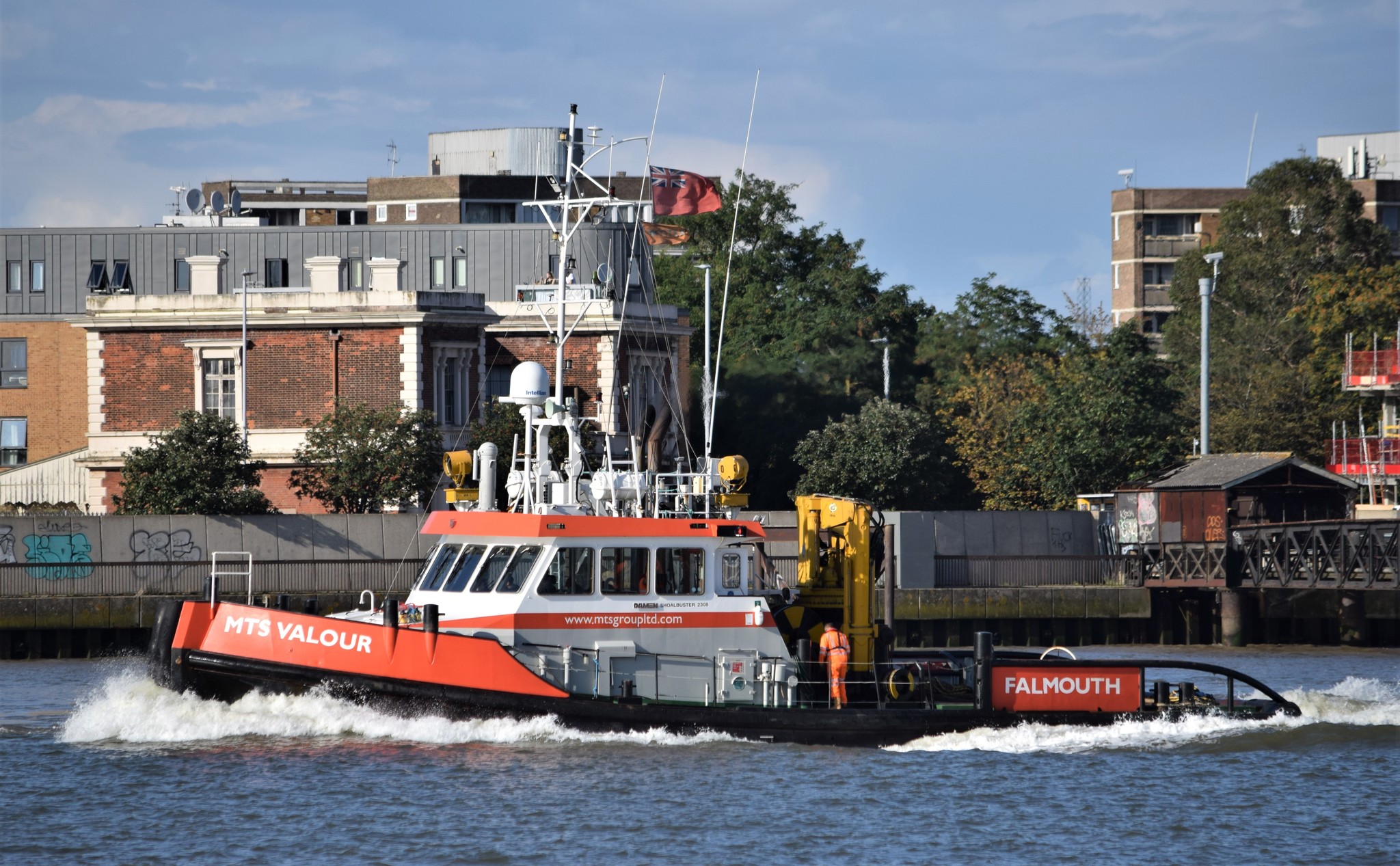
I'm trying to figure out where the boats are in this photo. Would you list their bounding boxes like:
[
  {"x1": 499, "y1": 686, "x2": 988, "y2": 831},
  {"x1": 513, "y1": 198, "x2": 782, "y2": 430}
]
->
[{"x1": 145, "y1": 78, "x2": 1303, "y2": 746}]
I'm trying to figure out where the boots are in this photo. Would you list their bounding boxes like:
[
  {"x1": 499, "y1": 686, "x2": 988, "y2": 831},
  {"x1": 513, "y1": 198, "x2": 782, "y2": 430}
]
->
[{"x1": 830, "y1": 697, "x2": 841, "y2": 709}]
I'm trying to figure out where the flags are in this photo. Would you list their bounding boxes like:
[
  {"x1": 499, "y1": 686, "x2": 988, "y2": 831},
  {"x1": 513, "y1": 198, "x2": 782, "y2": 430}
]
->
[
  {"x1": 649, "y1": 165, "x2": 721, "y2": 216},
  {"x1": 640, "y1": 221, "x2": 690, "y2": 246}
]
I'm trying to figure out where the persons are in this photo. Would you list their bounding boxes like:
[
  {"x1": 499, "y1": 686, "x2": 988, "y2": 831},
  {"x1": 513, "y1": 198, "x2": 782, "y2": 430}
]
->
[
  {"x1": 818, "y1": 622, "x2": 851, "y2": 710},
  {"x1": 639, "y1": 574, "x2": 647, "y2": 594},
  {"x1": 541, "y1": 271, "x2": 556, "y2": 284},
  {"x1": 566, "y1": 270, "x2": 576, "y2": 284}
]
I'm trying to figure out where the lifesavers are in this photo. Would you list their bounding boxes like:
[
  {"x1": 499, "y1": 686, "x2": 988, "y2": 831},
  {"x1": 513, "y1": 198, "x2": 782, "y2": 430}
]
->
[{"x1": 889, "y1": 668, "x2": 914, "y2": 698}]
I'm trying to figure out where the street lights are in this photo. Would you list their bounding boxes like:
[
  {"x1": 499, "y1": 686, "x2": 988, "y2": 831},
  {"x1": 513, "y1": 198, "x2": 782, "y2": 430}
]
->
[
  {"x1": 241, "y1": 267, "x2": 256, "y2": 492},
  {"x1": 693, "y1": 263, "x2": 711, "y2": 459},
  {"x1": 869, "y1": 337, "x2": 891, "y2": 402}
]
